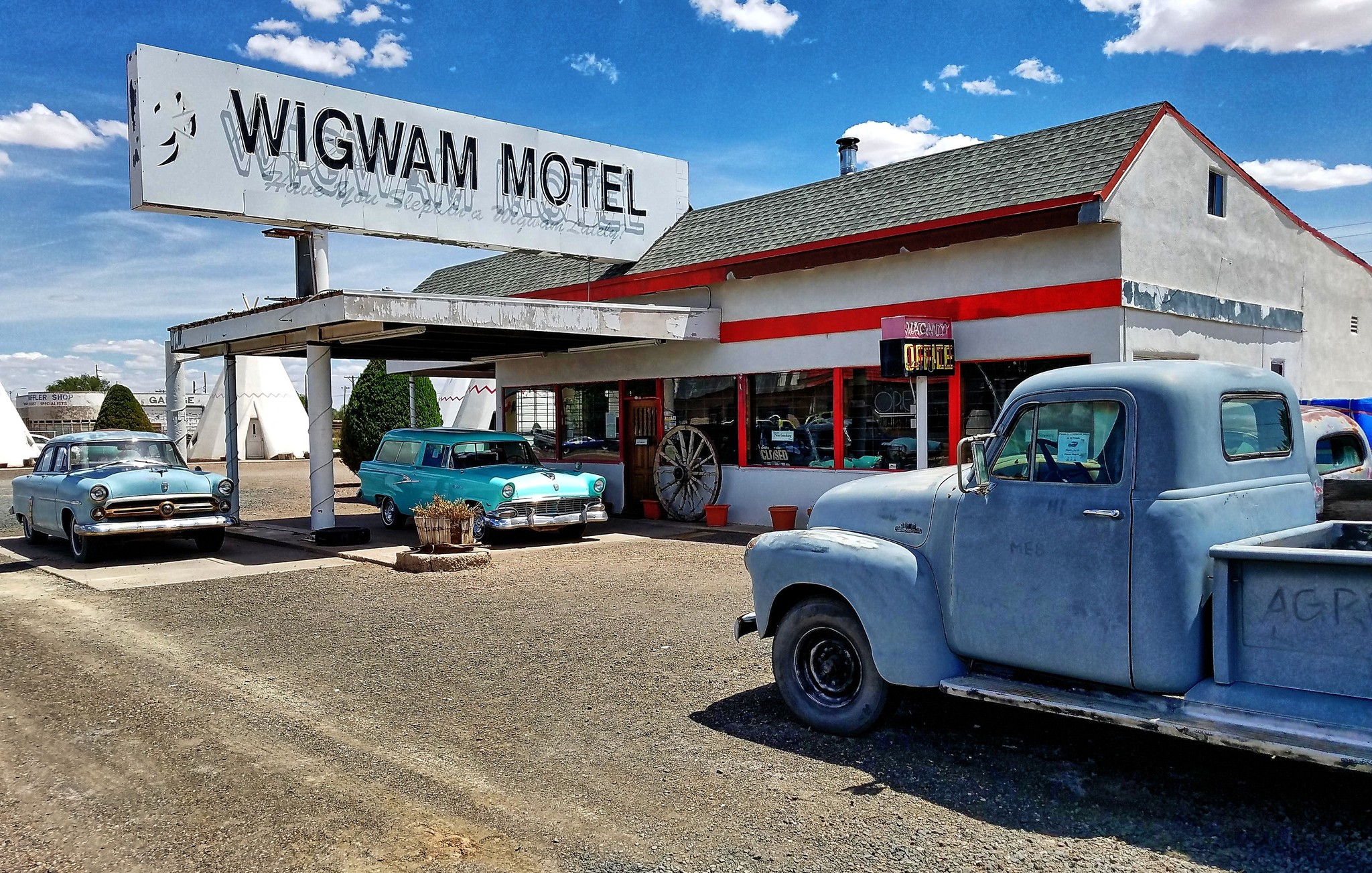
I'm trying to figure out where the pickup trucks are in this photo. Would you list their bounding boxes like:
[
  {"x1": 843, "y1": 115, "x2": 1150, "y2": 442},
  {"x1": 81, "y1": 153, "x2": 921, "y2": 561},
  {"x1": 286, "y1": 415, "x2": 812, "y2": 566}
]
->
[{"x1": 734, "y1": 358, "x2": 1372, "y2": 784}]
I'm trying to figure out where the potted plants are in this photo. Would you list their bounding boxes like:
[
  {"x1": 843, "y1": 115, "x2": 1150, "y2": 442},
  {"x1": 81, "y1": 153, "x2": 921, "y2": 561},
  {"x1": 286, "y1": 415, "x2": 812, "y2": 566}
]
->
[{"x1": 408, "y1": 493, "x2": 492, "y2": 552}]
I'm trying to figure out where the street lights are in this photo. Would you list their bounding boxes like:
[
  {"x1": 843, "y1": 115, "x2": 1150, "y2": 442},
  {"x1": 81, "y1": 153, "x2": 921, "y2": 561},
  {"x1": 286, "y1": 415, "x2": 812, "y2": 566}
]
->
[
  {"x1": 10, "y1": 387, "x2": 26, "y2": 401},
  {"x1": 341, "y1": 385, "x2": 349, "y2": 415}
]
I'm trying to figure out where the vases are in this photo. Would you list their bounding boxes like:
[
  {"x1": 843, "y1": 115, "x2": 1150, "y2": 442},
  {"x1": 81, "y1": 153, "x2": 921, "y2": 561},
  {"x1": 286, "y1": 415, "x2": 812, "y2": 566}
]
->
[
  {"x1": 769, "y1": 505, "x2": 798, "y2": 531},
  {"x1": 641, "y1": 499, "x2": 663, "y2": 517},
  {"x1": 704, "y1": 503, "x2": 731, "y2": 527}
]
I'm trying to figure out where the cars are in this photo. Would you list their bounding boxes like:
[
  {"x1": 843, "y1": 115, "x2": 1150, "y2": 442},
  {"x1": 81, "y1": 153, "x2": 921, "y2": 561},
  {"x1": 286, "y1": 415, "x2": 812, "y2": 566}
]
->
[
  {"x1": 562, "y1": 435, "x2": 609, "y2": 454},
  {"x1": 756, "y1": 416, "x2": 950, "y2": 470},
  {"x1": 11, "y1": 428, "x2": 237, "y2": 563},
  {"x1": 357, "y1": 425, "x2": 609, "y2": 542}
]
